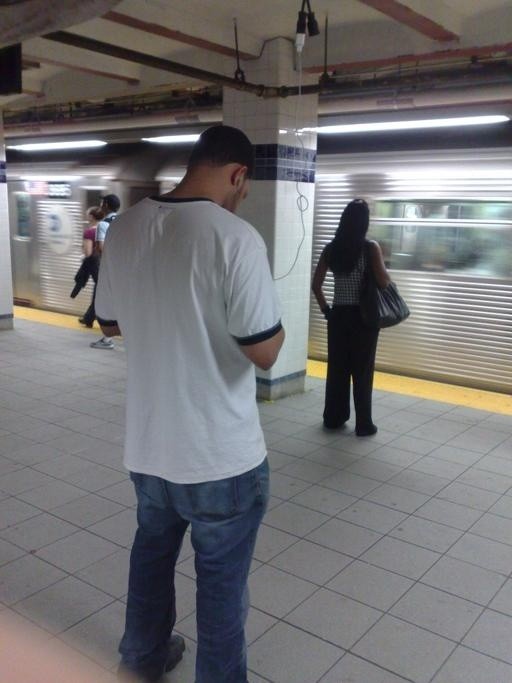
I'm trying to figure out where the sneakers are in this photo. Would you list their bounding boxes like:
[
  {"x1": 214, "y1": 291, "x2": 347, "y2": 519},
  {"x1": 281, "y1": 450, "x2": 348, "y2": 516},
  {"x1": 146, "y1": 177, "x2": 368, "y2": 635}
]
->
[
  {"x1": 116, "y1": 635, "x2": 185, "y2": 683},
  {"x1": 78, "y1": 317, "x2": 93, "y2": 328},
  {"x1": 90, "y1": 337, "x2": 114, "y2": 349}
]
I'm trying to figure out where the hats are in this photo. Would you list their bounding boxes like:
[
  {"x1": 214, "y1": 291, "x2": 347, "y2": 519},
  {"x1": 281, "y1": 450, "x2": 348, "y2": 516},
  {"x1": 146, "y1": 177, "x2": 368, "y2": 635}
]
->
[{"x1": 98, "y1": 194, "x2": 120, "y2": 209}]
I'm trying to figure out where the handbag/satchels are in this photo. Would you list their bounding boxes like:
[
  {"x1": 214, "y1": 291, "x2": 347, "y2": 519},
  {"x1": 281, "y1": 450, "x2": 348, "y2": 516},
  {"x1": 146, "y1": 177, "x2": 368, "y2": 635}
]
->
[{"x1": 358, "y1": 280, "x2": 411, "y2": 329}]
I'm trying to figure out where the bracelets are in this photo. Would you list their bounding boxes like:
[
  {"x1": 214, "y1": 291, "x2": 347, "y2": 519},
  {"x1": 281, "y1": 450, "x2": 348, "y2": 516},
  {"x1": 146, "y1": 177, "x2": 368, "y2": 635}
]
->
[{"x1": 321, "y1": 305, "x2": 330, "y2": 315}]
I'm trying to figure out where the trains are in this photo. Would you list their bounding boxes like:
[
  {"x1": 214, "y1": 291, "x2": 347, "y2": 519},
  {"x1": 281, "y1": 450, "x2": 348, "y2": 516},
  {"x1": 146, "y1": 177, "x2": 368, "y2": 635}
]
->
[{"x1": 5, "y1": 145, "x2": 511, "y2": 397}]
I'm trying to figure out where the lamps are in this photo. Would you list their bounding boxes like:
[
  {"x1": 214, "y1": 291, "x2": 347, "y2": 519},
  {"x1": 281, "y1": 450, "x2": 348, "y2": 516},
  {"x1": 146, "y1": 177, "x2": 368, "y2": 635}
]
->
[{"x1": 294, "y1": 1, "x2": 322, "y2": 54}]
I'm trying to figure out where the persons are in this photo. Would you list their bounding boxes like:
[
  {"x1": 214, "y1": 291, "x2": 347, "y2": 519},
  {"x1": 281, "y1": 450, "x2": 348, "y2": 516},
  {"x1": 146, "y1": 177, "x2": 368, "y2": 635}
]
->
[
  {"x1": 94, "y1": 126, "x2": 286, "y2": 683},
  {"x1": 78, "y1": 205, "x2": 105, "y2": 327},
  {"x1": 312, "y1": 199, "x2": 390, "y2": 436},
  {"x1": 89, "y1": 194, "x2": 120, "y2": 350}
]
